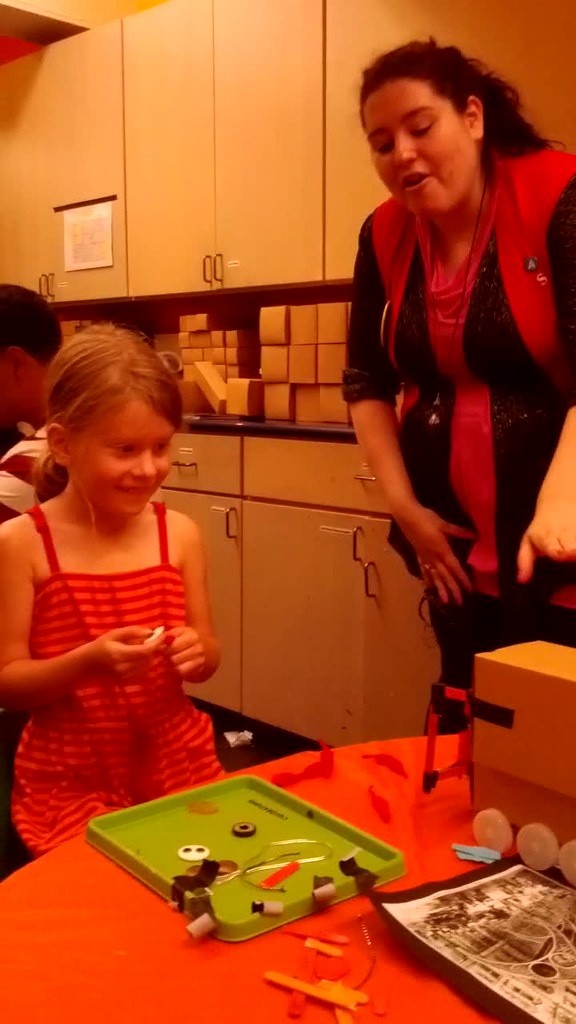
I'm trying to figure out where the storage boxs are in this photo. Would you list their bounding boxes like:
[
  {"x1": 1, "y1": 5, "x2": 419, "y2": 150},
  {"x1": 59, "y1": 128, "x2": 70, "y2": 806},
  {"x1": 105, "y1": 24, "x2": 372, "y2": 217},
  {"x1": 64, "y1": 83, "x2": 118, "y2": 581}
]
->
[
  {"x1": 471, "y1": 639, "x2": 576, "y2": 799},
  {"x1": 59, "y1": 301, "x2": 350, "y2": 423},
  {"x1": 473, "y1": 762, "x2": 576, "y2": 845}
]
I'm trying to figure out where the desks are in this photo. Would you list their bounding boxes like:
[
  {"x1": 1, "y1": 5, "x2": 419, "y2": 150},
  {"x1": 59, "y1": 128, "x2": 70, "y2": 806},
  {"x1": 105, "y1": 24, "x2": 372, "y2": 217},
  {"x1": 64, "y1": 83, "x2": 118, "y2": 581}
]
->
[{"x1": 0, "y1": 734, "x2": 502, "y2": 1024}]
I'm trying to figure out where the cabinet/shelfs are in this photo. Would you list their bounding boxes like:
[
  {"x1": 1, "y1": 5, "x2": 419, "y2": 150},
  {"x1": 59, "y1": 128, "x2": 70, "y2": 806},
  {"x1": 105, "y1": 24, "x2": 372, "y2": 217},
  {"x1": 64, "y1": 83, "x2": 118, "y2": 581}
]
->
[
  {"x1": 149, "y1": 431, "x2": 244, "y2": 720},
  {"x1": 122, "y1": 0, "x2": 326, "y2": 302},
  {"x1": 0, "y1": 20, "x2": 131, "y2": 309},
  {"x1": 241, "y1": 437, "x2": 442, "y2": 747}
]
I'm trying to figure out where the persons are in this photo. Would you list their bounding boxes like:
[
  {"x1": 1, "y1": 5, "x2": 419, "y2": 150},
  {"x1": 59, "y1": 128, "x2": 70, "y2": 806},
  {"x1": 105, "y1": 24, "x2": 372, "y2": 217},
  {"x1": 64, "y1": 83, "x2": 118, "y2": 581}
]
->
[
  {"x1": 0, "y1": 321, "x2": 228, "y2": 860},
  {"x1": 0, "y1": 280, "x2": 64, "y2": 522},
  {"x1": 341, "y1": 36, "x2": 576, "y2": 732}
]
